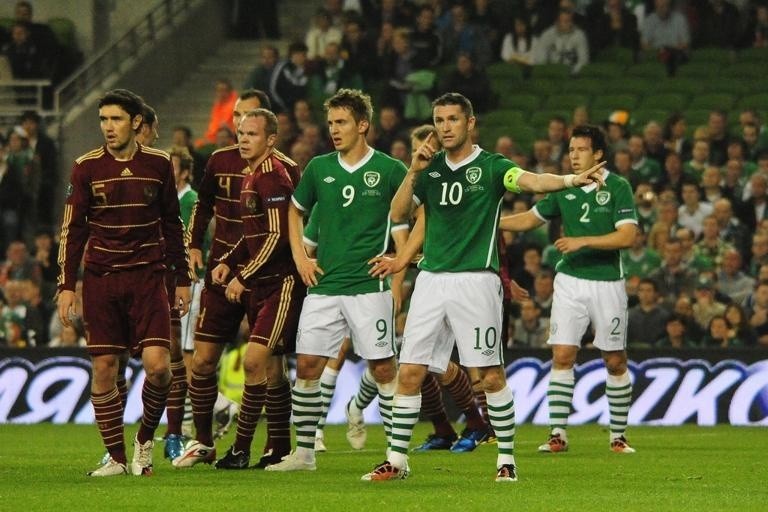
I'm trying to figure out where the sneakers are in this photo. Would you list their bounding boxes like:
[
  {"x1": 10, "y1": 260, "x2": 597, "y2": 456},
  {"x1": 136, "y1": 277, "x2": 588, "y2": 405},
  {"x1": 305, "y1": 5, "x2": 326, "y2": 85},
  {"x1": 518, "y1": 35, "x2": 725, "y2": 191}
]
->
[
  {"x1": 165, "y1": 437, "x2": 181, "y2": 459},
  {"x1": 250, "y1": 449, "x2": 291, "y2": 468},
  {"x1": 312, "y1": 431, "x2": 326, "y2": 451},
  {"x1": 132, "y1": 434, "x2": 154, "y2": 475},
  {"x1": 412, "y1": 434, "x2": 460, "y2": 450},
  {"x1": 346, "y1": 397, "x2": 367, "y2": 449},
  {"x1": 213, "y1": 448, "x2": 251, "y2": 469},
  {"x1": 362, "y1": 462, "x2": 410, "y2": 480},
  {"x1": 87, "y1": 458, "x2": 129, "y2": 478},
  {"x1": 609, "y1": 438, "x2": 636, "y2": 453},
  {"x1": 264, "y1": 455, "x2": 317, "y2": 473},
  {"x1": 495, "y1": 465, "x2": 518, "y2": 482},
  {"x1": 214, "y1": 402, "x2": 240, "y2": 439},
  {"x1": 171, "y1": 439, "x2": 215, "y2": 468},
  {"x1": 537, "y1": 437, "x2": 569, "y2": 452},
  {"x1": 449, "y1": 428, "x2": 491, "y2": 452}
]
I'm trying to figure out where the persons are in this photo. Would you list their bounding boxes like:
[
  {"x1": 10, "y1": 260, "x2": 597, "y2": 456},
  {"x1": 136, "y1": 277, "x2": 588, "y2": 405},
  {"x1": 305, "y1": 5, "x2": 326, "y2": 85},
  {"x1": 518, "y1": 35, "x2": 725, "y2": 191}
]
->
[
  {"x1": 509, "y1": 269, "x2": 554, "y2": 317},
  {"x1": 299, "y1": 191, "x2": 411, "y2": 457},
  {"x1": 579, "y1": 2, "x2": 767, "y2": 79},
  {"x1": 166, "y1": 147, "x2": 243, "y2": 444},
  {"x1": 511, "y1": 299, "x2": 552, "y2": 350},
  {"x1": 506, "y1": 321, "x2": 524, "y2": 348},
  {"x1": 212, "y1": 109, "x2": 306, "y2": 469},
  {"x1": 301, "y1": 123, "x2": 329, "y2": 158},
  {"x1": 264, "y1": 89, "x2": 411, "y2": 479},
  {"x1": 293, "y1": 98, "x2": 318, "y2": 135},
  {"x1": 374, "y1": 107, "x2": 406, "y2": 155},
  {"x1": 288, "y1": 140, "x2": 316, "y2": 177},
  {"x1": 406, "y1": 123, "x2": 492, "y2": 455},
  {"x1": 20, "y1": 109, "x2": 55, "y2": 229},
  {"x1": 195, "y1": 126, "x2": 235, "y2": 163},
  {"x1": 172, "y1": 91, "x2": 301, "y2": 468},
  {"x1": 367, "y1": 120, "x2": 379, "y2": 149},
  {"x1": 6, "y1": 23, "x2": 54, "y2": 107},
  {"x1": 494, "y1": 118, "x2": 567, "y2": 238},
  {"x1": 390, "y1": 139, "x2": 411, "y2": 172},
  {"x1": 56, "y1": 89, "x2": 191, "y2": 479},
  {"x1": 568, "y1": 99, "x2": 609, "y2": 147},
  {"x1": 2, "y1": 0, "x2": 59, "y2": 53},
  {"x1": 0, "y1": 231, "x2": 57, "y2": 349},
  {"x1": 197, "y1": 80, "x2": 244, "y2": 144},
  {"x1": 14, "y1": 126, "x2": 37, "y2": 198},
  {"x1": 243, "y1": 2, "x2": 587, "y2": 108},
  {"x1": 272, "y1": 110, "x2": 293, "y2": 152},
  {"x1": 610, "y1": 106, "x2": 767, "y2": 355},
  {"x1": 467, "y1": 364, "x2": 498, "y2": 440},
  {"x1": 163, "y1": 123, "x2": 198, "y2": 163},
  {"x1": 498, "y1": 125, "x2": 640, "y2": 454},
  {"x1": 101, "y1": 103, "x2": 195, "y2": 466},
  {"x1": 510, "y1": 245, "x2": 556, "y2": 299},
  {"x1": 361, "y1": 92, "x2": 609, "y2": 484}
]
